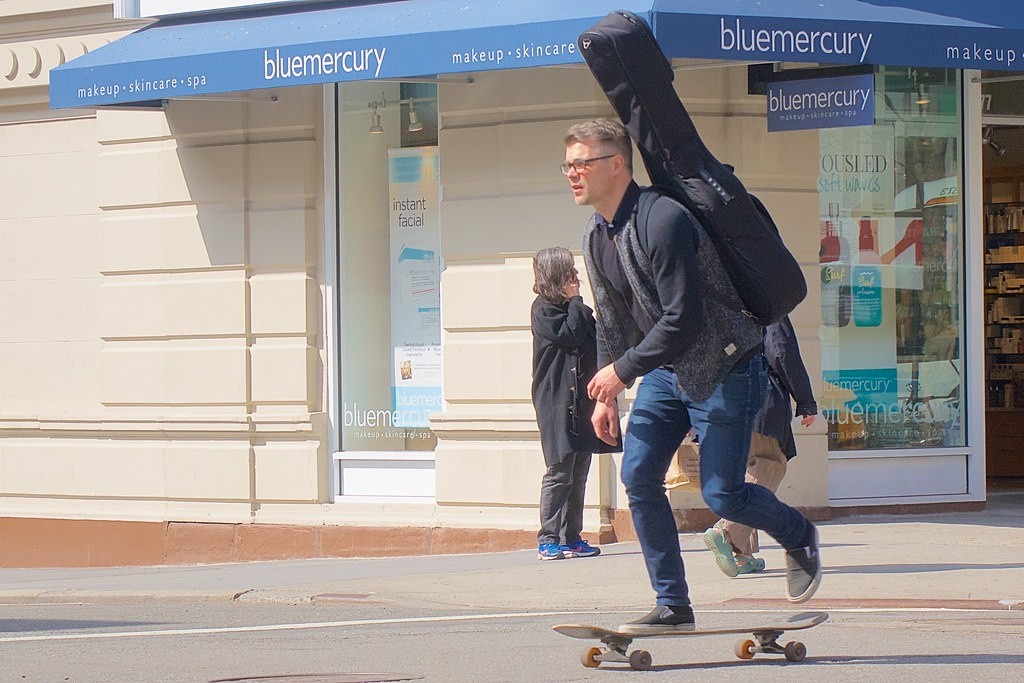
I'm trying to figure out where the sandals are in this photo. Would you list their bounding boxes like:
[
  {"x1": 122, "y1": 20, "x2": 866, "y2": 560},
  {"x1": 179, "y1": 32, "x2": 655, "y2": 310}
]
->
[{"x1": 703, "y1": 525, "x2": 765, "y2": 577}]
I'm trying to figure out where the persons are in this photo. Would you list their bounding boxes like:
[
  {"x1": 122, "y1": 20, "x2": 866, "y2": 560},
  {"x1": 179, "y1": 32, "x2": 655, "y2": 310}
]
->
[
  {"x1": 564, "y1": 118, "x2": 822, "y2": 633},
  {"x1": 531, "y1": 247, "x2": 601, "y2": 561},
  {"x1": 702, "y1": 314, "x2": 817, "y2": 577}
]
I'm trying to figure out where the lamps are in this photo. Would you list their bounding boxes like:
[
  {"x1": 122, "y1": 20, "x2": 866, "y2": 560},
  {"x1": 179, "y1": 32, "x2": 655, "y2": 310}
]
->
[
  {"x1": 369, "y1": 100, "x2": 384, "y2": 134},
  {"x1": 408, "y1": 97, "x2": 423, "y2": 131}
]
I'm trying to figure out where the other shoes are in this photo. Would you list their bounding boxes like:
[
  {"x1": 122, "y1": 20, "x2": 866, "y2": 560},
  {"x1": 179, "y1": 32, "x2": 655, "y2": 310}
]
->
[
  {"x1": 619, "y1": 605, "x2": 695, "y2": 633},
  {"x1": 786, "y1": 518, "x2": 822, "y2": 603}
]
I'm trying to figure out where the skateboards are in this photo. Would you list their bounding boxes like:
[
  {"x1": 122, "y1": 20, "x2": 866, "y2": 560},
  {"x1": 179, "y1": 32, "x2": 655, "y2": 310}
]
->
[{"x1": 552, "y1": 609, "x2": 831, "y2": 671}]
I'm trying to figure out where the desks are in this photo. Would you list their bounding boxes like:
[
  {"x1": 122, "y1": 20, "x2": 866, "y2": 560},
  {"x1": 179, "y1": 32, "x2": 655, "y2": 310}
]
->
[{"x1": 983, "y1": 232, "x2": 1024, "y2": 477}]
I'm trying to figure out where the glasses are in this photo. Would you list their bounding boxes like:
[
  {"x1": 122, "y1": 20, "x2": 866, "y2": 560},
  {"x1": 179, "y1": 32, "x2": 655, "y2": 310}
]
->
[{"x1": 560, "y1": 154, "x2": 615, "y2": 175}]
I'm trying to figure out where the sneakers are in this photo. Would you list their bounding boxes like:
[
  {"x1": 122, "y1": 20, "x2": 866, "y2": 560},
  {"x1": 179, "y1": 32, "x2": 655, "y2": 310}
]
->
[{"x1": 537, "y1": 539, "x2": 600, "y2": 560}]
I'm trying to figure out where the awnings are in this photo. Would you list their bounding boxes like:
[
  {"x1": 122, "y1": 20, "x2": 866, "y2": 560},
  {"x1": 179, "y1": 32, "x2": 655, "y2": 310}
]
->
[{"x1": 47, "y1": 0, "x2": 1024, "y2": 111}]
